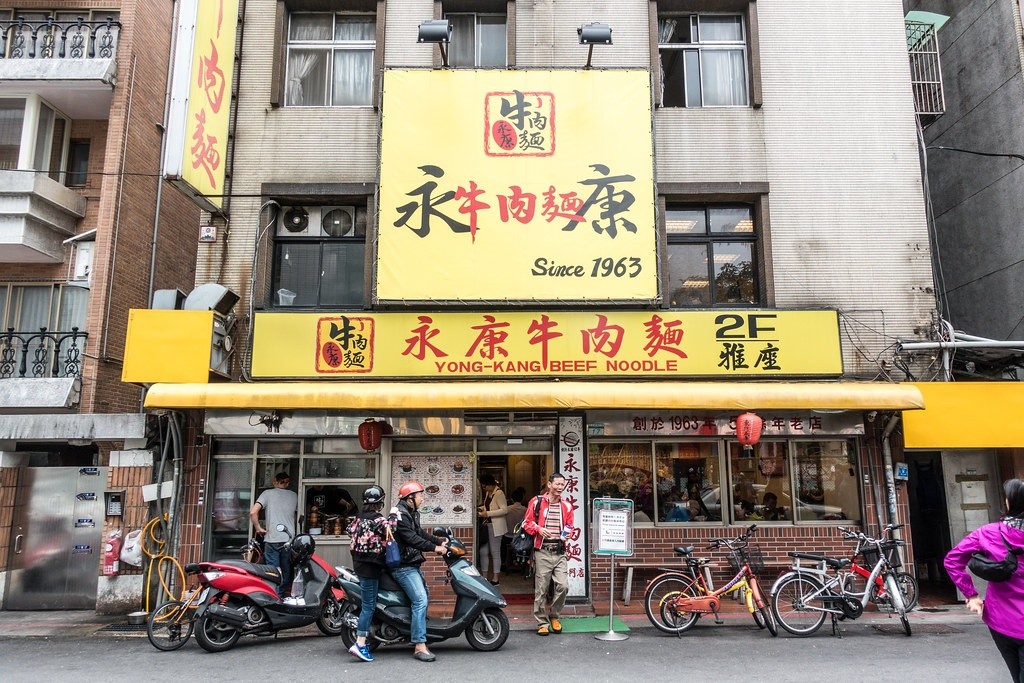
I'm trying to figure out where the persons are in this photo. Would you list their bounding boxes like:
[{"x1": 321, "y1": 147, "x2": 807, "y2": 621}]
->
[
  {"x1": 944, "y1": 479, "x2": 1024, "y2": 683},
  {"x1": 476, "y1": 478, "x2": 546, "y2": 570},
  {"x1": 306, "y1": 484, "x2": 358, "y2": 518},
  {"x1": 523, "y1": 473, "x2": 574, "y2": 635},
  {"x1": 588, "y1": 469, "x2": 824, "y2": 523},
  {"x1": 477, "y1": 474, "x2": 508, "y2": 587},
  {"x1": 348, "y1": 485, "x2": 400, "y2": 662},
  {"x1": 251, "y1": 471, "x2": 298, "y2": 598},
  {"x1": 387, "y1": 480, "x2": 449, "y2": 661}
]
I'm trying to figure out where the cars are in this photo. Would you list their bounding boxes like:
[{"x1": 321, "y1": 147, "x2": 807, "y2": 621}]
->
[{"x1": 675, "y1": 483, "x2": 843, "y2": 521}]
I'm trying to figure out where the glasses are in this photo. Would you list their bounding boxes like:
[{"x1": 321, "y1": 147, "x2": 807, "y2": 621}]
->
[{"x1": 483, "y1": 485, "x2": 487, "y2": 489}]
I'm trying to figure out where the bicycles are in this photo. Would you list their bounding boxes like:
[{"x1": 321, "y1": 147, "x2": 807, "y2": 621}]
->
[
  {"x1": 147, "y1": 539, "x2": 259, "y2": 652},
  {"x1": 770, "y1": 524, "x2": 920, "y2": 642},
  {"x1": 644, "y1": 522, "x2": 777, "y2": 642}
]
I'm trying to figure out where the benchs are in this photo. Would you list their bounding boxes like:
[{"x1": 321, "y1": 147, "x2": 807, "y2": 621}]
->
[
  {"x1": 619, "y1": 562, "x2": 718, "y2": 607},
  {"x1": 730, "y1": 559, "x2": 832, "y2": 605}
]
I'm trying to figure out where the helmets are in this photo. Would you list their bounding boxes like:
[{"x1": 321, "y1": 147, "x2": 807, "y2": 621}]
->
[
  {"x1": 362, "y1": 485, "x2": 385, "y2": 506},
  {"x1": 288, "y1": 534, "x2": 316, "y2": 566},
  {"x1": 398, "y1": 482, "x2": 424, "y2": 498}
]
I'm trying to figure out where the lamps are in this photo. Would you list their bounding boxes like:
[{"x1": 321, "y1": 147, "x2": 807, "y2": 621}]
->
[
  {"x1": 578, "y1": 21, "x2": 614, "y2": 66},
  {"x1": 415, "y1": 19, "x2": 455, "y2": 67}
]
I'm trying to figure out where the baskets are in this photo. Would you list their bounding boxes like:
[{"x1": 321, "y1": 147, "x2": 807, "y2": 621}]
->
[
  {"x1": 726, "y1": 543, "x2": 763, "y2": 574},
  {"x1": 860, "y1": 542, "x2": 902, "y2": 570}
]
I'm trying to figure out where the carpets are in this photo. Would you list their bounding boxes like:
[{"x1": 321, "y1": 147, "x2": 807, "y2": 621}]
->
[{"x1": 548, "y1": 614, "x2": 631, "y2": 634}]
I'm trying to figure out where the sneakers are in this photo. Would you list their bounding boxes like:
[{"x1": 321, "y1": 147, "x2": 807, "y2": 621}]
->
[
  {"x1": 348, "y1": 642, "x2": 375, "y2": 662},
  {"x1": 413, "y1": 647, "x2": 436, "y2": 661},
  {"x1": 490, "y1": 580, "x2": 500, "y2": 587},
  {"x1": 538, "y1": 627, "x2": 549, "y2": 636},
  {"x1": 550, "y1": 618, "x2": 562, "y2": 633}
]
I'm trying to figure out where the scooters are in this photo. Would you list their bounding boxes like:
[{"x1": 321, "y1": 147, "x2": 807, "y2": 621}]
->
[
  {"x1": 331, "y1": 525, "x2": 510, "y2": 652},
  {"x1": 193, "y1": 513, "x2": 358, "y2": 650}
]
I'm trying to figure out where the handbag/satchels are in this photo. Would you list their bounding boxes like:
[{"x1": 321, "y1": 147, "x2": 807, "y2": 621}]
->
[
  {"x1": 383, "y1": 524, "x2": 401, "y2": 564},
  {"x1": 511, "y1": 495, "x2": 543, "y2": 556},
  {"x1": 291, "y1": 568, "x2": 306, "y2": 599},
  {"x1": 966, "y1": 527, "x2": 1018, "y2": 583}
]
